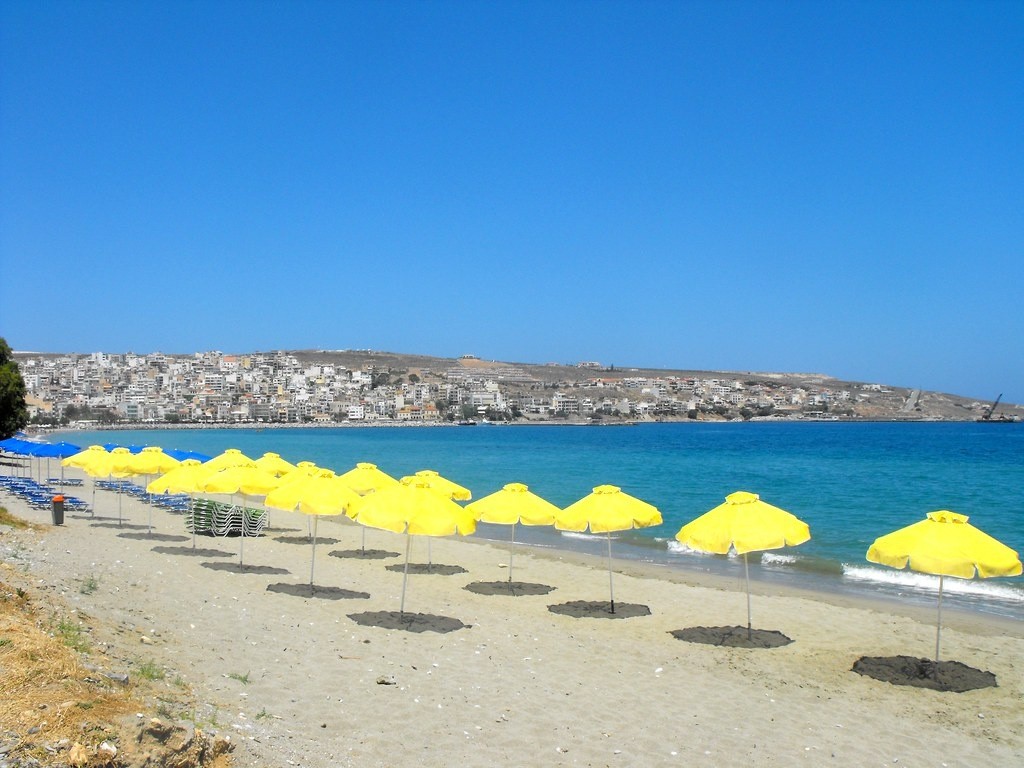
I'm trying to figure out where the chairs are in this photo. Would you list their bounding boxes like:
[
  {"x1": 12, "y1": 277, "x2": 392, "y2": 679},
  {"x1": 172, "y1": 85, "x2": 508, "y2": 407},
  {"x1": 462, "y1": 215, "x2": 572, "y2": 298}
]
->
[
  {"x1": 0, "y1": 474, "x2": 90, "y2": 512},
  {"x1": 95, "y1": 480, "x2": 269, "y2": 539}
]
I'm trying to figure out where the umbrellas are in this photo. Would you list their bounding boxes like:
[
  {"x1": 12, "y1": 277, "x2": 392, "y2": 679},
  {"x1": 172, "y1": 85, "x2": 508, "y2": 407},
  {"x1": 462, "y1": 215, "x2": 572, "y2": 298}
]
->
[
  {"x1": 554, "y1": 484, "x2": 663, "y2": 613},
  {"x1": 255, "y1": 452, "x2": 339, "y2": 540},
  {"x1": 464, "y1": 483, "x2": 561, "y2": 587},
  {"x1": 145, "y1": 449, "x2": 280, "y2": 569},
  {"x1": 340, "y1": 462, "x2": 400, "y2": 558},
  {"x1": 400, "y1": 470, "x2": 472, "y2": 570},
  {"x1": 60, "y1": 443, "x2": 213, "y2": 533},
  {"x1": 347, "y1": 482, "x2": 476, "y2": 617},
  {"x1": 264, "y1": 478, "x2": 360, "y2": 590},
  {"x1": 866, "y1": 510, "x2": 1022, "y2": 678},
  {"x1": 675, "y1": 491, "x2": 811, "y2": 639},
  {"x1": 0, "y1": 432, "x2": 81, "y2": 493}
]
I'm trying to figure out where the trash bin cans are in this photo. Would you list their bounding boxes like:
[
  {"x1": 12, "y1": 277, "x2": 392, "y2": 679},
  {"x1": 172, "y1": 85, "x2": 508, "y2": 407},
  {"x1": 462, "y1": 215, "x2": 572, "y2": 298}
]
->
[{"x1": 52, "y1": 495, "x2": 64, "y2": 524}]
